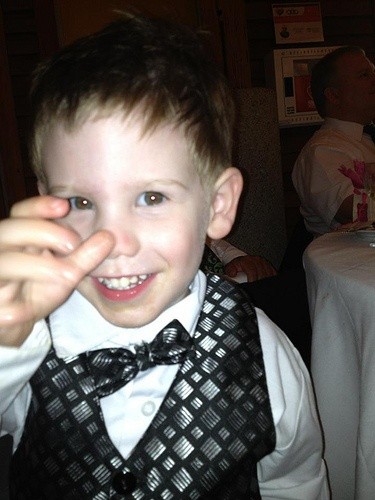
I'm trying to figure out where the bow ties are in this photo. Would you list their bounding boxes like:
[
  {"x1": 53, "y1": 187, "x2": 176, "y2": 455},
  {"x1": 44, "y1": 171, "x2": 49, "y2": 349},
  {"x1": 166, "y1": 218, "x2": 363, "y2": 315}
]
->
[{"x1": 85, "y1": 319, "x2": 196, "y2": 399}]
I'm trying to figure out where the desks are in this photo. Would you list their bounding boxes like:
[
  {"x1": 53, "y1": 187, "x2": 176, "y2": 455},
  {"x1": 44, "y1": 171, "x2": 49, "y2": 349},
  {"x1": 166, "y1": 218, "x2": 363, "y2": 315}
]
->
[{"x1": 304, "y1": 232, "x2": 375, "y2": 500}]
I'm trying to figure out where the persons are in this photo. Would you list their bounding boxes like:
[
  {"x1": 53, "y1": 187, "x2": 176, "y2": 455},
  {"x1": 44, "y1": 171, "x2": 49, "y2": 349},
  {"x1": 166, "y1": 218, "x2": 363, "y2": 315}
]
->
[
  {"x1": 200, "y1": 45, "x2": 375, "y2": 284},
  {"x1": 1, "y1": 15, "x2": 331, "y2": 500}
]
